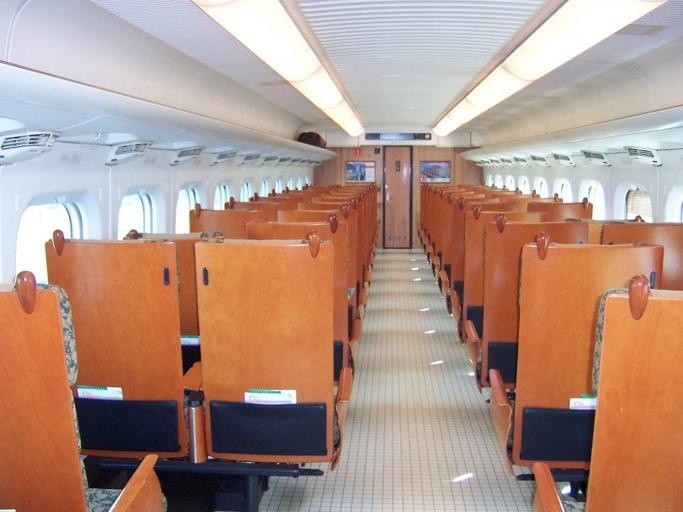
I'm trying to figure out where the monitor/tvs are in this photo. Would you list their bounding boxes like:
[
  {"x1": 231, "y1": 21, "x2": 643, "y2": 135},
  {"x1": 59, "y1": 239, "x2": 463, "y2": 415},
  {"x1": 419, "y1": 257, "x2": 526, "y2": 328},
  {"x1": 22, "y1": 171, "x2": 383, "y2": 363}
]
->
[
  {"x1": 345, "y1": 161, "x2": 376, "y2": 182},
  {"x1": 419, "y1": 161, "x2": 451, "y2": 183}
]
